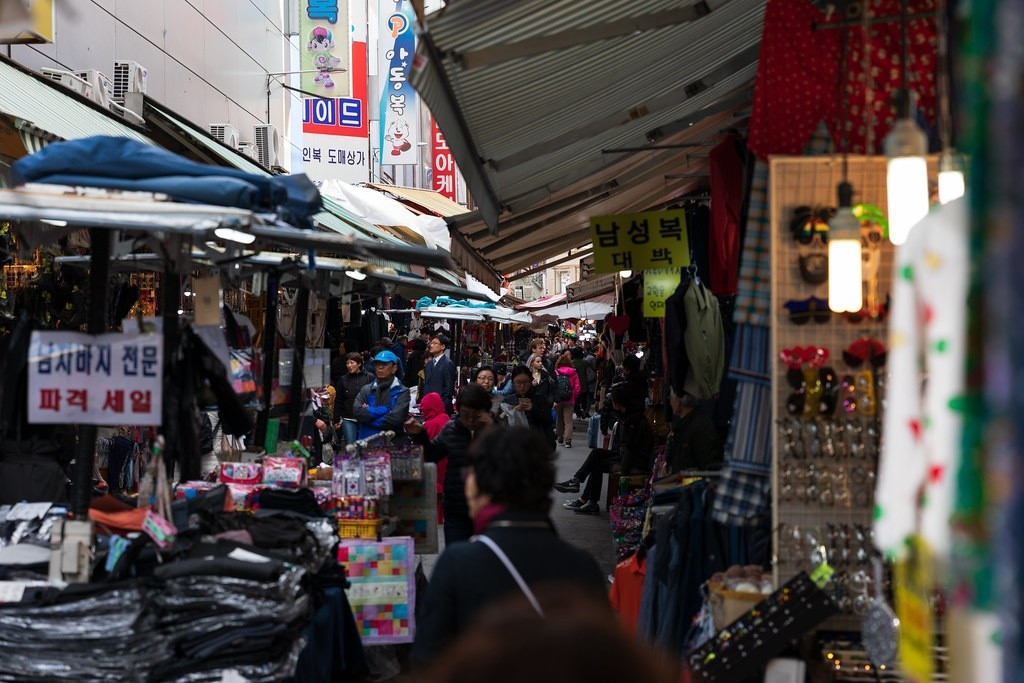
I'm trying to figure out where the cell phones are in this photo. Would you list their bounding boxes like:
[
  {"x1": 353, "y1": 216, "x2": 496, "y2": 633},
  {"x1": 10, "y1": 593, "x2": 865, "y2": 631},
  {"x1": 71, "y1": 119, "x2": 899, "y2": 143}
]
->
[{"x1": 519, "y1": 398, "x2": 528, "y2": 404}]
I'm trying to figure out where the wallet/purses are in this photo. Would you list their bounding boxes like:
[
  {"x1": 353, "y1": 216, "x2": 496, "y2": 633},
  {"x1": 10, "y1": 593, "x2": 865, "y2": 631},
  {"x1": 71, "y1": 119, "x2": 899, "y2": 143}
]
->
[
  {"x1": 220, "y1": 462, "x2": 262, "y2": 485},
  {"x1": 263, "y1": 458, "x2": 306, "y2": 488}
]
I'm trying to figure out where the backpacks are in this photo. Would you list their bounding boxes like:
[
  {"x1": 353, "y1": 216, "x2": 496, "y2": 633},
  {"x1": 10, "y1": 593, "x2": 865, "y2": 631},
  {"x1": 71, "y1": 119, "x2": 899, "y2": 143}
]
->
[
  {"x1": 555, "y1": 369, "x2": 576, "y2": 400},
  {"x1": 199, "y1": 413, "x2": 221, "y2": 455}
]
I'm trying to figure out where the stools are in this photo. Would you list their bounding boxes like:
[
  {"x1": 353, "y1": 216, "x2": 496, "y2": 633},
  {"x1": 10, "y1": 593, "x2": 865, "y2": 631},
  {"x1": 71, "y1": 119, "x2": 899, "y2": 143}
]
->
[{"x1": 605, "y1": 473, "x2": 648, "y2": 513}]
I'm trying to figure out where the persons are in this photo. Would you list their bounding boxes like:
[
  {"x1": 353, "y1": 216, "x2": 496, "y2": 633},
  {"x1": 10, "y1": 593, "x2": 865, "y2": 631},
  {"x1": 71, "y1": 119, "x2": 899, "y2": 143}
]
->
[
  {"x1": 299, "y1": 321, "x2": 721, "y2": 550},
  {"x1": 417, "y1": 424, "x2": 675, "y2": 683}
]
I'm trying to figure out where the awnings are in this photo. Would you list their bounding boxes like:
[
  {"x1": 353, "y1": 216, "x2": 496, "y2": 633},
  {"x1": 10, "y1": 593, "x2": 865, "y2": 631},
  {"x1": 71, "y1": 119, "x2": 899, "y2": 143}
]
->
[{"x1": 0, "y1": 0, "x2": 765, "y2": 334}]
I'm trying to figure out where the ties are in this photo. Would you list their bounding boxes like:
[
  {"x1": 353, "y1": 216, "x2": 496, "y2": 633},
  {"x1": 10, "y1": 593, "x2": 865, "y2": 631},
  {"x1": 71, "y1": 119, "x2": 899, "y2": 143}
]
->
[{"x1": 431, "y1": 360, "x2": 436, "y2": 370}]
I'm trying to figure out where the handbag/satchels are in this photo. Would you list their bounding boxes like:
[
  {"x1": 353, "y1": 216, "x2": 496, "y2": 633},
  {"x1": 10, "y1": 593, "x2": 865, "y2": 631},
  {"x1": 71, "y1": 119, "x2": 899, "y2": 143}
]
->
[
  {"x1": 337, "y1": 539, "x2": 416, "y2": 646},
  {"x1": 322, "y1": 443, "x2": 334, "y2": 465}
]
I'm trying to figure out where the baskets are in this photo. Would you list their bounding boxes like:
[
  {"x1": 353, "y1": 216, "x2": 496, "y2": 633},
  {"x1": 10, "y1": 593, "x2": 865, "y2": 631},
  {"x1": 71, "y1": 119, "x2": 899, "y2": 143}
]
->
[
  {"x1": 338, "y1": 519, "x2": 384, "y2": 540},
  {"x1": 685, "y1": 569, "x2": 835, "y2": 683}
]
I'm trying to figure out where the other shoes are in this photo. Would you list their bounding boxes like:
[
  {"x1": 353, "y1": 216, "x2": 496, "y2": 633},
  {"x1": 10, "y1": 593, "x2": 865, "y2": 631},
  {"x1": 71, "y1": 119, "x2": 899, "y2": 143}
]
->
[
  {"x1": 585, "y1": 413, "x2": 591, "y2": 418},
  {"x1": 577, "y1": 415, "x2": 585, "y2": 419},
  {"x1": 563, "y1": 499, "x2": 585, "y2": 510},
  {"x1": 559, "y1": 440, "x2": 563, "y2": 445},
  {"x1": 565, "y1": 442, "x2": 571, "y2": 447}
]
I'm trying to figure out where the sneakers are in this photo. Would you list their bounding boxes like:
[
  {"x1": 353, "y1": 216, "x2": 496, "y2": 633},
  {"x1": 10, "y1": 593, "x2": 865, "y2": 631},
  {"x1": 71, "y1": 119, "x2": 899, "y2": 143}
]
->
[
  {"x1": 574, "y1": 500, "x2": 600, "y2": 515},
  {"x1": 554, "y1": 480, "x2": 580, "y2": 493}
]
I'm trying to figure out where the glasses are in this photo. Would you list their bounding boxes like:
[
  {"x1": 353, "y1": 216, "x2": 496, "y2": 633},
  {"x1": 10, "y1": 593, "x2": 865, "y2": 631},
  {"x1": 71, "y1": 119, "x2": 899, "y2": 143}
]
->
[
  {"x1": 430, "y1": 342, "x2": 439, "y2": 344},
  {"x1": 477, "y1": 376, "x2": 495, "y2": 382},
  {"x1": 826, "y1": 640, "x2": 894, "y2": 679},
  {"x1": 776, "y1": 297, "x2": 894, "y2": 617},
  {"x1": 514, "y1": 379, "x2": 531, "y2": 384}
]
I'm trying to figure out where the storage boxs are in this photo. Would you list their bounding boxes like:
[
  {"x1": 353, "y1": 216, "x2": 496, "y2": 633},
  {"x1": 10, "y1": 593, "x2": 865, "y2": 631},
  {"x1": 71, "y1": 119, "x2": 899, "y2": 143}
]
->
[{"x1": 338, "y1": 516, "x2": 383, "y2": 540}]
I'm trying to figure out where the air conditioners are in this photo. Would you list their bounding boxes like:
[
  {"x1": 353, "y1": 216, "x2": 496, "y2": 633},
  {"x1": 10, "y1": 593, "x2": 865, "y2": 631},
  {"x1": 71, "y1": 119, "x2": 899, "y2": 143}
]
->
[
  {"x1": 112, "y1": 58, "x2": 147, "y2": 99},
  {"x1": 41, "y1": 67, "x2": 111, "y2": 109},
  {"x1": 254, "y1": 123, "x2": 279, "y2": 172},
  {"x1": 209, "y1": 125, "x2": 240, "y2": 150},
  {"x1": 237, "y1": 141, "x2": 259, "y2": 162}
]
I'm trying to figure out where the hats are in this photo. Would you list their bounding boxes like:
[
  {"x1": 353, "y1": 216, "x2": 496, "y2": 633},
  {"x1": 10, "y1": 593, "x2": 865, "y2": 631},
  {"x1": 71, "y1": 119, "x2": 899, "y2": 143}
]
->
[
  {"x1": 372, "y1": 350, "x2": 396, "y2": 364},
  {"x1": 670, "y1": 379, "x2": 689, "y2": 400}
]
